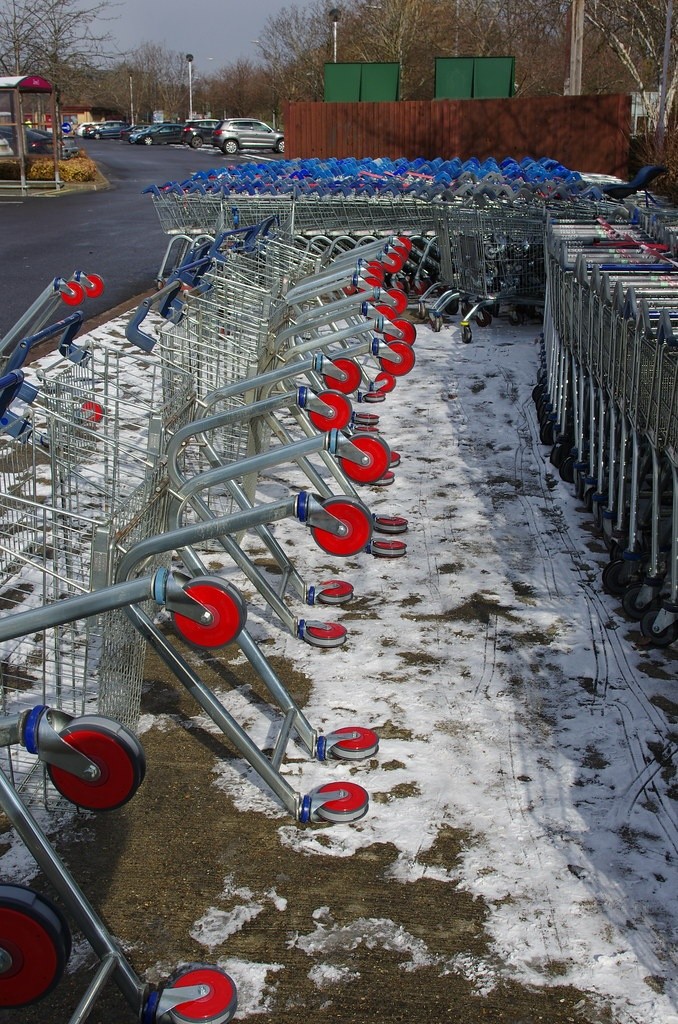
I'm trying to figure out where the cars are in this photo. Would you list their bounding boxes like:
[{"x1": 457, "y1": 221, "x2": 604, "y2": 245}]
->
[
  {"x1": 130, "y1": 122, "x2": 169, "y2": 145},
  {"x1": 136, "y1": 124, "x2": 188, "y2": 146},
  {"x1": 83, "y1": 123, "x2": 103, "y2": 137},
  {"x1": 89, "y1": 122, "x2": 129, "y2": 139},
  {"x1": 120, "y1": 125, "x2": 147, "y2": 144},
  {"x1": 0, "y1": 125, "x2": 79, "y2": 160}
]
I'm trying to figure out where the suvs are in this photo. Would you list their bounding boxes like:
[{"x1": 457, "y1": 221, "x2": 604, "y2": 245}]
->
[
  {"x1": 213, "y1": 117, "x2": 284, "y2": 154},
  {"x1": 74, "y1": 122, "x2": 97, "y2": 137},
  {"x1": 180, "y1": 119, "x2": 238, "y2": 148}
]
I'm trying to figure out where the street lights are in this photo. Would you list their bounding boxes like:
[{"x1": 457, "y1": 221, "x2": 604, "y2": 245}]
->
[
  {"x1": 186, "y1": 54, "x2": 194, "y2": 120},
  {"x1": 128, "y1": 68, "x2": 135, "y2": 128},
  {"x1": 251, "y1": 40, "x2": 276, "y2": 129},
  {"x1": 209, "y1": 57, "x2": 227, "y2": 119},
  {"x1": 327, "y1": 8, "x2": 342, "y2": 62}
]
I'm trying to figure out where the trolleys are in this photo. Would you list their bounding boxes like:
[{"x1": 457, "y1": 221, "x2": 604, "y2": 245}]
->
[
  {"x1": 142, "y1": 153, "x2": 678, "y2": 343},
  {"x1": 530, "y1": 208, "x2": 678, "y2": 645},
  {"x1": 1, "y1": 214, "x2": 416, "y2": 1023}
]
